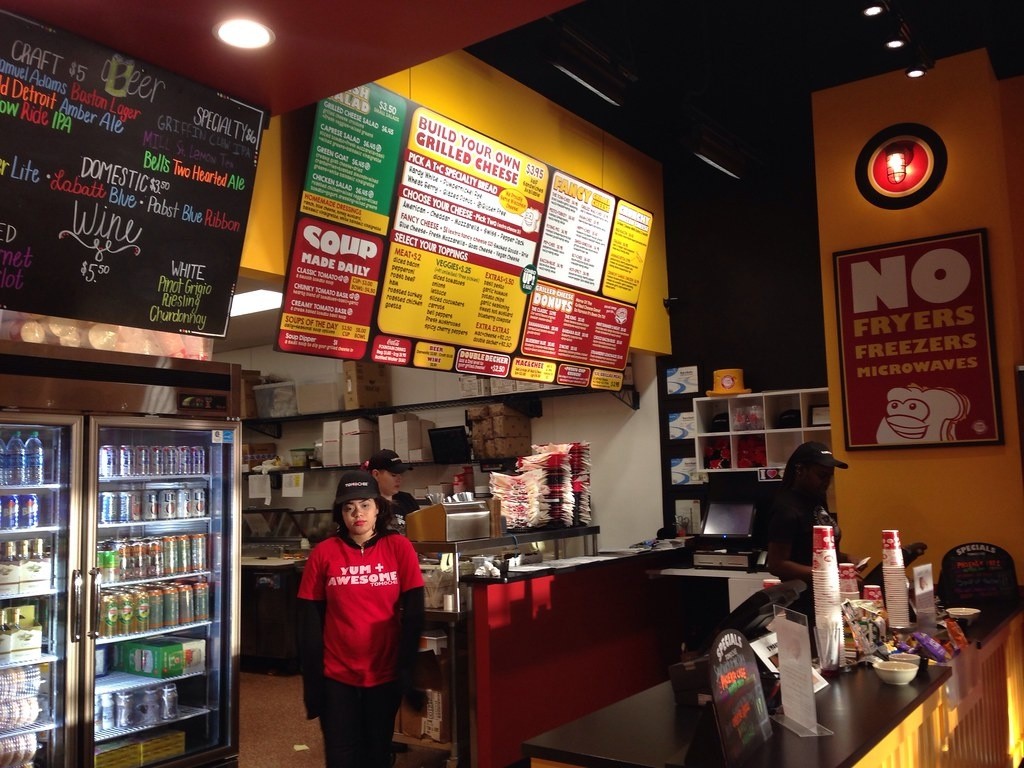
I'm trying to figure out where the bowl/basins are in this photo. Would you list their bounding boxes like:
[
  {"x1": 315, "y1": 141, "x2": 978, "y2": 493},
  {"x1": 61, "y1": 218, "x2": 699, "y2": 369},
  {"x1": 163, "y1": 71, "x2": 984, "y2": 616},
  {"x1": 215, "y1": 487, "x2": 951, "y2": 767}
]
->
[
  {"x1": 289, "y1": 449, "x2": 315, "y2": 466},
  {"x1": 870, "y1": 662, "x2": 919, "y2": 686},
  {"x1": 887, "y1": 654, "x2": 921, "y2": 666},
  {"x1": 946, "y1": 607, "x2": 981, "y2": 628}
]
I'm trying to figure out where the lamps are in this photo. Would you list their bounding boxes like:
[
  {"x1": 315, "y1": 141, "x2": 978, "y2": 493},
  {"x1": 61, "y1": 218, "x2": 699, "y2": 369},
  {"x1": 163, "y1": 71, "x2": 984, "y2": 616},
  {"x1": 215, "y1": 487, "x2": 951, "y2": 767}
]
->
[
  {"x1": 858, "y1": 0, "x2": 886, "y2": 19},
  {"x1": 544, "y1": 37, "x2": 626, "y2": 107},
  {"x1": 880, "y1": 17, "x2": 908, "y2": 51},
  {"x1": 905, "y1": 48, "x2": 926, "y2": 78},
  {"x1": 886, "y1": 145, "x2": 914, "y2": 184},
  {"x1": 683, "y1": 124, "x2": 746, "y2": 180}
]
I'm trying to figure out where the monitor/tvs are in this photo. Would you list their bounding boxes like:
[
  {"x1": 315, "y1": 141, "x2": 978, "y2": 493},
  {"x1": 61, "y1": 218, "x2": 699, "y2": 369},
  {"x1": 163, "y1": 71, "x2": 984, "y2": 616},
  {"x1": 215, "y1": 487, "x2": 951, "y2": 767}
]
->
[
  {"x1": 699, "y1": 499, "x2": 758, "y2": 550},
  {"x1": 858, "y1": 543, "x2": 927, "y2": 594},
  {"x1": 709, "y1": 579, "x2": 809, "y2": 653}
]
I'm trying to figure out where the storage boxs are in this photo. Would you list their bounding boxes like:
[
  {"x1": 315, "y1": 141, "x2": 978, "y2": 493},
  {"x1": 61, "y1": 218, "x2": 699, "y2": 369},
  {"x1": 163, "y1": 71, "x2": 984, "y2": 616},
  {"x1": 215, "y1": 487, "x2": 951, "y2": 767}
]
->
[
  {"x1": 240, "y1": 351, "x2": 633, "y2": 503},
  {"x1": 395, "y1": 638, "x2": 450, "y2": 742}
]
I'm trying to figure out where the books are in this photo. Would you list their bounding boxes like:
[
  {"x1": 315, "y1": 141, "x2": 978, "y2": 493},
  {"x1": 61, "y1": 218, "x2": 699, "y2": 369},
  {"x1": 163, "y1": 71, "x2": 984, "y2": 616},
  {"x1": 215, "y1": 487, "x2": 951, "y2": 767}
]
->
[{"x1": 598, "y1": 538, "x2": 685, "y2": 553}]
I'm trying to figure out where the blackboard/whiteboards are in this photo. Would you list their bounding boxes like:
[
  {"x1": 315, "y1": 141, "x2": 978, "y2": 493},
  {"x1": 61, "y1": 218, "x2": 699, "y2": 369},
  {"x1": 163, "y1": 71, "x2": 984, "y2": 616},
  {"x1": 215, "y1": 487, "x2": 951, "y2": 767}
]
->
[{"x1": 0, "y1": 16, "x2": 270, "y2": 337}]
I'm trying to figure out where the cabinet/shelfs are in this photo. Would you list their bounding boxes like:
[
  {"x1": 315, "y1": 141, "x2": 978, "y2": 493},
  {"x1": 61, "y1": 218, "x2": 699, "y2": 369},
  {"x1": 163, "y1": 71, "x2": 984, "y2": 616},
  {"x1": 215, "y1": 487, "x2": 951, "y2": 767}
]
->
[
  {"x1": 240, "y1": 386, "x2": 639, "y2": 479},
  {"x1": 646, "y1": 569, "x2": 780, "y2": 680},
  {"x1": 694, "y1": 387, "x2": 831, "y2": 473},
  {"x1": 391, "y1": 526, "x2": 600, "y2": 768}
]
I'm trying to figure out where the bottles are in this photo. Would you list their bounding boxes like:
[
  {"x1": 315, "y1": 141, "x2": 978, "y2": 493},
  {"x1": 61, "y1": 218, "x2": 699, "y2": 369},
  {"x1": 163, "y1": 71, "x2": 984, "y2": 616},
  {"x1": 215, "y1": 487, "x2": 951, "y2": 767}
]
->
[
  {"x1": 0, "y1": 428, "x2": 46, "y2": 485},
  {"x1": 92, "y1": 685, "x2": 178, "y2": 730}
]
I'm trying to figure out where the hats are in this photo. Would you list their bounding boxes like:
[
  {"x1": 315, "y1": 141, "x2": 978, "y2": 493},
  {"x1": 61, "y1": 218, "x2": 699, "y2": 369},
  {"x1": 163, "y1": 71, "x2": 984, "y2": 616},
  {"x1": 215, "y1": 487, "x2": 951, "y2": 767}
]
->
[
  {"x1": 367, "y1": 448, "x2": 414, "y2": 475},
  {"x1": 791, "y1": 440, "x2": 849, "y2": 471},
  {"x1": 334, "y1": 468, "x2": 380, "y2": 504}
]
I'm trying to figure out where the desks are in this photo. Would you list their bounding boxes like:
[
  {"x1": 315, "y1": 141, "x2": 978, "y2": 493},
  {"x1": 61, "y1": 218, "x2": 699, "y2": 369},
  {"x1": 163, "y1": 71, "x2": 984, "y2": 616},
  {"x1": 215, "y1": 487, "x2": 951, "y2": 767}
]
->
[{"x1": 520, "y1": 583, "x2": 1024, "y2": 768}]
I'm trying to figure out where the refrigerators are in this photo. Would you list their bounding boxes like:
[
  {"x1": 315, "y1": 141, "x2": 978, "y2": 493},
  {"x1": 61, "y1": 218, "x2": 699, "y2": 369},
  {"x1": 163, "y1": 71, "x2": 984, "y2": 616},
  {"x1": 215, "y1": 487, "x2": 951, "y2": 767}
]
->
[{"x1": 0, "y1": 338, "x2": 243, "y2": 767}]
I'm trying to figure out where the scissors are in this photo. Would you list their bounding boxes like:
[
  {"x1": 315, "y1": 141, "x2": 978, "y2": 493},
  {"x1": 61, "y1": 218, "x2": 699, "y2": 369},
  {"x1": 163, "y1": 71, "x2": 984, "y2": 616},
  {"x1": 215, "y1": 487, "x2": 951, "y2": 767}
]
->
[{"x1": 678, "y1": 516, "x2": 689, "y2": 524}]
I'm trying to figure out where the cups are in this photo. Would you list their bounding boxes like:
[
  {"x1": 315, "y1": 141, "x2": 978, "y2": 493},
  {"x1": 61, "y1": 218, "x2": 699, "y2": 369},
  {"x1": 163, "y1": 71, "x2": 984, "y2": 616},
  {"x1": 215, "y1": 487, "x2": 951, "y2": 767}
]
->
[
  {"x1": 813, "y1": 524, "x2": 909, "y2": 675},
  {"x1": 763, "y1": 579, "x2": 782, "y2": 590},
  {"x1": 732, "y1": 405, "x2": 764, "y2": 431}
]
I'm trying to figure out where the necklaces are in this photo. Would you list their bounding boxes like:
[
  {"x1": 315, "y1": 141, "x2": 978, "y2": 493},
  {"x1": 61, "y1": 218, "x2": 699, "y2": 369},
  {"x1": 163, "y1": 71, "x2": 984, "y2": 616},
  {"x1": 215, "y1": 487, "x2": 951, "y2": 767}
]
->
[{"x1": 355, "y1": 533, "x2": 379, "y2": 555}]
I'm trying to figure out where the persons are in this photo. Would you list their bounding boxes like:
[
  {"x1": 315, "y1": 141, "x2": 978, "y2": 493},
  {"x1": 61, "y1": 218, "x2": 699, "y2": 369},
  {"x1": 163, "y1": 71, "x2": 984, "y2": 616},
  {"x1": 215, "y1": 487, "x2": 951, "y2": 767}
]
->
[
  {"x1": 361, "y1": 448, "x2": 421, "y2": 534},
  {"x1": 295, "y1": 471, "x2": 425, "y2": 768},
  {"x1": 767, "y1": 442, "x2": 867, "y2": 625}
]
